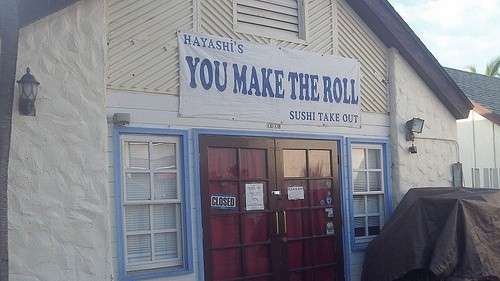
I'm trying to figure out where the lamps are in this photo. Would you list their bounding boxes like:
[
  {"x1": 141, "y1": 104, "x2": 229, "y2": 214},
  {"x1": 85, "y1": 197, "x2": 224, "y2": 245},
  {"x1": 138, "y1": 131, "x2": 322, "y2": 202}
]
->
[
  {"x1": 18, "y1": 67, "x2": 40, "y2": 118},
  {"x1": 406, "y1": 116, "x2": 424, "y2": 143}
]
